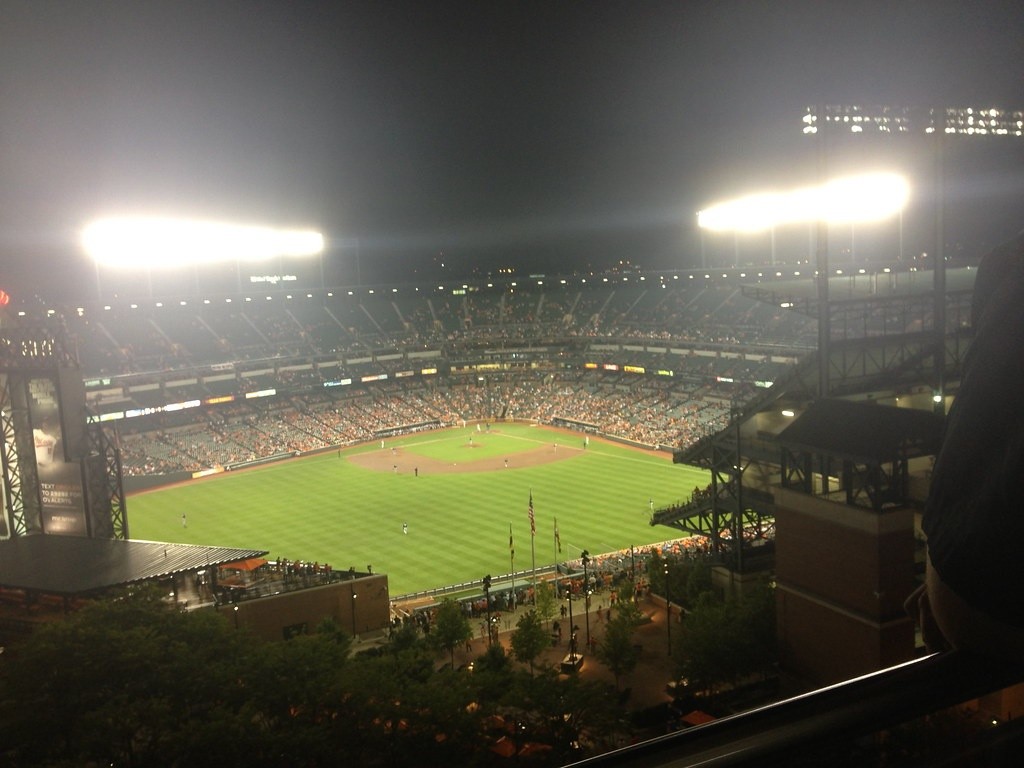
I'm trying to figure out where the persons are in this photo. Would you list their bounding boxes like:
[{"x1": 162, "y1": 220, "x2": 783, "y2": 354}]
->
[
  {"x1": 81, "y1": 281, "x2": 820, "y2": 768},
  {"x1": 904, "y1": 233, "x2": 1023, "y2": 668}
]
